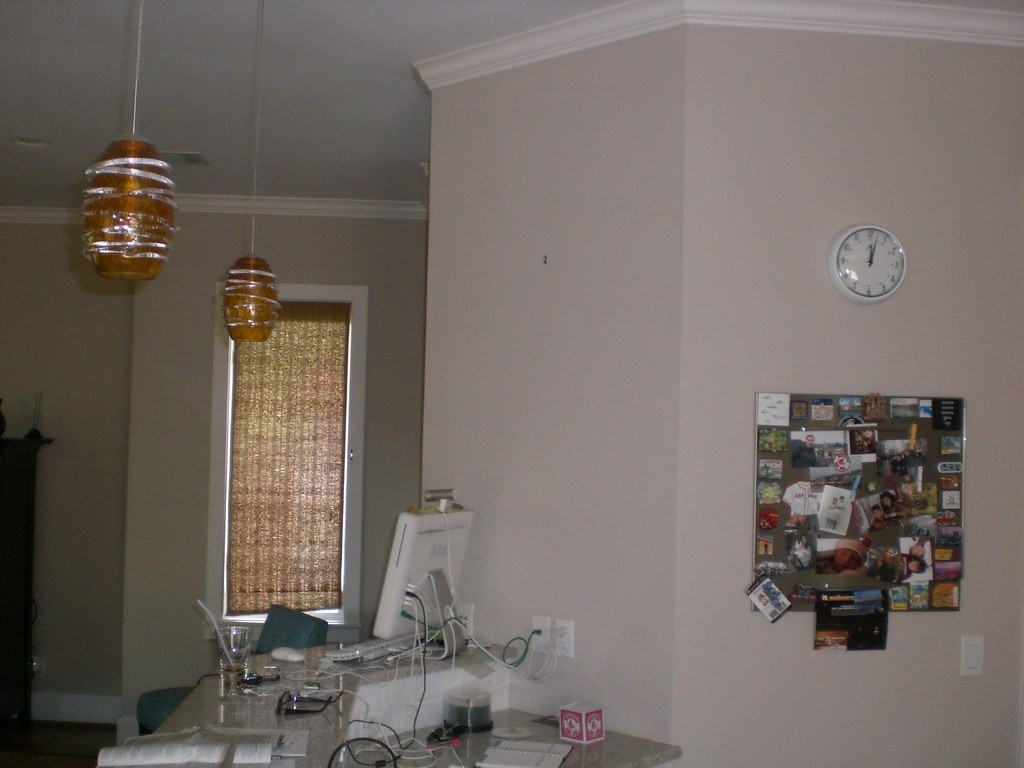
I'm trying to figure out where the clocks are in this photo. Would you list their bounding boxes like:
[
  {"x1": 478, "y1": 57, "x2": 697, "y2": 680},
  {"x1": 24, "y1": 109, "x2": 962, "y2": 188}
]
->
[{"x1": 823, "y1": 224, "x2": 906, "y2": 306}]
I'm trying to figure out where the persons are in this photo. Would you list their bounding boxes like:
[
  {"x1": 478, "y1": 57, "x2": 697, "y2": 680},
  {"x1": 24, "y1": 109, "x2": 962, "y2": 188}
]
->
[
  {"x1": 898, "y1": 537, "x2": 930, "y2": 583},
  {"x1": 819, "y1": 539, "x2": 869, "y2": 578},
  {"x1": 891, "y1": 451, "x2": 912, "y2": 476},
  {"x1": 792, "y1": 434, "x2": 819, "y2": 468},
  {"x1": 868, "y1": 491, "x2": 904, "y2": 533},
  {"x1": 789, "y1": 535, "x2": 813, "y2": 568}
]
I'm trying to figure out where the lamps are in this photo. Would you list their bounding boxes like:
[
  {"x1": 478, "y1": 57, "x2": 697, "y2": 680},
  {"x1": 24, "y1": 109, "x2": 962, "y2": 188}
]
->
[
  {"x1": 221, "y1": 1, "x2": 281, "y2": 342},
  {"x1": 81, "y1": 0, "x2": 180, "y2": 281}
]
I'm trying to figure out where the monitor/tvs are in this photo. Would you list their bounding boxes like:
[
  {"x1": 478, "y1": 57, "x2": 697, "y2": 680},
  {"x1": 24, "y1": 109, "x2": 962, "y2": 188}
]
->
[{"x1": 372, "y1": 507, "x2": 465, "y2": 660}]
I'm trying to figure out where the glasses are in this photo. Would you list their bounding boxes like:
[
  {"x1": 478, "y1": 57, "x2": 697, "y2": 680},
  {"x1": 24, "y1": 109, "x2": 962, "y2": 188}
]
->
[{"x1": 275, "y1": 689, "x2": 343, "y2": 715}]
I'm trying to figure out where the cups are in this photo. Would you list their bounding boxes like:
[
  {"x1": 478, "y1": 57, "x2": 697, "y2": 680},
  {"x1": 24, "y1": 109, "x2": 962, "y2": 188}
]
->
[{"x1": 217, "y1": 626, "x2": 251, "y2": 675}]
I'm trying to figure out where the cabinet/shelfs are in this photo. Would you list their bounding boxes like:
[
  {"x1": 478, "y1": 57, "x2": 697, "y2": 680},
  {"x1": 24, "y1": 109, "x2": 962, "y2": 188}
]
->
[{"x1": 0, "y1": 397, "x2": 53, "y2": 753}]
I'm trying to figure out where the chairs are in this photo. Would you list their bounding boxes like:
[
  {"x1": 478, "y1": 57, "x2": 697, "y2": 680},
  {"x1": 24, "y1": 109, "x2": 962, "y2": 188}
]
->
[{"x1": 136, "y1": 605, "x2": 328, "y2": 735}]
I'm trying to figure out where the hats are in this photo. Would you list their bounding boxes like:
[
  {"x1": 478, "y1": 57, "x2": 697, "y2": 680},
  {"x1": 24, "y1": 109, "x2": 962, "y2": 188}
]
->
[{"x1": 834, "y1": 539, "x2": 869, "y2": 565}]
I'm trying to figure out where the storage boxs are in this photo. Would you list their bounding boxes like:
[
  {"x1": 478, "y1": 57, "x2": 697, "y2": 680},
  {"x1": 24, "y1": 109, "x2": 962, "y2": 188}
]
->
[{"x1": 559, "y1": 700, "x2": 605, "y2": 743}]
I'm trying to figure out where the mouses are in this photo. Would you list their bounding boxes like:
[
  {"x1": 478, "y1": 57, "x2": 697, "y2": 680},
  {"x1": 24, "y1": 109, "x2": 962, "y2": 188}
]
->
[{"x1": 271, "y1": 648, "x2": 305, "y2": 662}]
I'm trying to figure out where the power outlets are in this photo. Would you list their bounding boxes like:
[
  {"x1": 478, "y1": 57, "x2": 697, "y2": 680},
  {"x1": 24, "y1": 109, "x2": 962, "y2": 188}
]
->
[
  {"x1": 532, "y1": 616, "x2": 550, "y2": 654},
  {"x1": 554, "y1": 619, "x2": 574, "y2": 658}
]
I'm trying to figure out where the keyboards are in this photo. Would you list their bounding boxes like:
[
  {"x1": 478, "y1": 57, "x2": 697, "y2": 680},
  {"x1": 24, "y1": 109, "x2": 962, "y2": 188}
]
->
[{"x1": 324, "y1": 633, "x2": 429, "y2": 660}]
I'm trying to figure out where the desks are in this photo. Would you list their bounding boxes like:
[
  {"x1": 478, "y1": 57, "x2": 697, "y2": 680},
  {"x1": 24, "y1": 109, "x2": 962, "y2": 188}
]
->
[{"x1": 134, "y1": 642, "x2": 682, "y2": 768}]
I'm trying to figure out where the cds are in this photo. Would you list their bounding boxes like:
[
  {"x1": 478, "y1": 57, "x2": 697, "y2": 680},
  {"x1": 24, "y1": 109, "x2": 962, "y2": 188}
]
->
[
  {"x1": 445, "y1": 699, "x2": 491, "y2": 729},
  {"x1": 492, "y1": 727, "x2": 534, "y2": 739}
]
